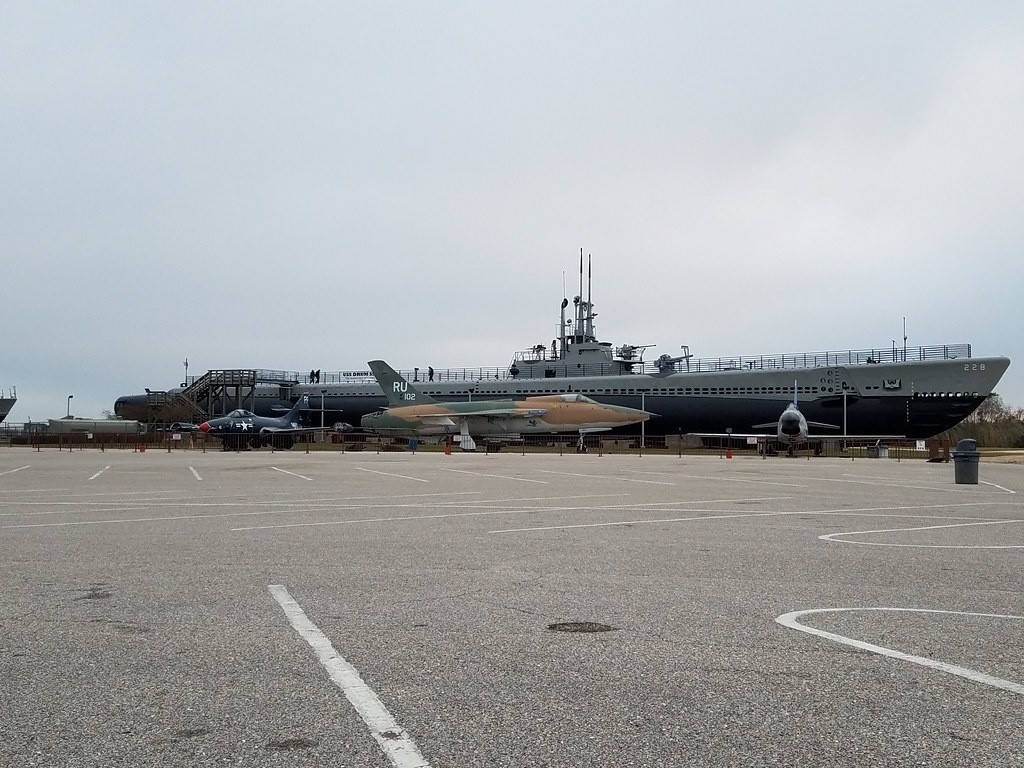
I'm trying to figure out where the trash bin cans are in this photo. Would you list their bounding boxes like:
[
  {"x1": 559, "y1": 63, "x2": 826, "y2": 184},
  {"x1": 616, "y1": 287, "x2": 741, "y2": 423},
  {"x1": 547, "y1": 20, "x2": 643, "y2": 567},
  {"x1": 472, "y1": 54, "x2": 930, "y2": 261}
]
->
[{"x1": 951, "y1": 437, "x2": 981, "y2": 485}]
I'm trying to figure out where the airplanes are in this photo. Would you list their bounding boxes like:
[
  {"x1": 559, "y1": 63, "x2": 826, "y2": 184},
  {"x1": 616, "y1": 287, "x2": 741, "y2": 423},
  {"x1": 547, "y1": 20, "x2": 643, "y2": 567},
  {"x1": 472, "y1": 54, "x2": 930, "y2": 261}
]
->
[
  {"x1": 156, "y1": 421, "x2": 199, "y2": 432},
  {"x1": 686, "y1": 379, "x2": 906, "y2": 457},
  {"x1": 352, "y1": 360, "x2": 662, "y2": 452},
  {"x1": 199, "y1": 392, "x2": 344, "y2": 451}
]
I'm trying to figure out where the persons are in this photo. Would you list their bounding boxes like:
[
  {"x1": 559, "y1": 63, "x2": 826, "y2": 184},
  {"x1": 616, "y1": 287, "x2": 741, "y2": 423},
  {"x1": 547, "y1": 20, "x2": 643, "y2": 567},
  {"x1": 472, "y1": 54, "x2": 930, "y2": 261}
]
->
[
  {"x1": 428, "y1": 366, "x2": 434, "y2": 382},
  {"x1": 315, "y1": 368, "x2": 321, "y2": 384},
  {"x1": 309, "y1": 369, "x2": 315, "y2": 384}
]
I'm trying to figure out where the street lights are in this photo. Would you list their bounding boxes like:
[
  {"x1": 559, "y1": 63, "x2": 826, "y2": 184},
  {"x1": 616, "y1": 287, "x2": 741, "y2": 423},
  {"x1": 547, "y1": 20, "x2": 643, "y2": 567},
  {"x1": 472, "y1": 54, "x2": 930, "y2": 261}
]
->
[
  {"x1": 842, "y1": 386, "x2": 850, "y2": 451},
  {"x1": 321, "y1": 389, "x2": 327, "y2": 442},
  {"x1": 68, "y1": 396, "x2": 73, "y2": 419}
]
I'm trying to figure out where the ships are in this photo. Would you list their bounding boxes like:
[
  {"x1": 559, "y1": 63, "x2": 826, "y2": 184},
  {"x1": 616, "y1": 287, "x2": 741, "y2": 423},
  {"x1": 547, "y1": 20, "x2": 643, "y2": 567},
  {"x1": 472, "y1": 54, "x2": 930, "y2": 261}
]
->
[{"x1": 114, "y1": 247, "x2": 1010, "y2": 440}]
